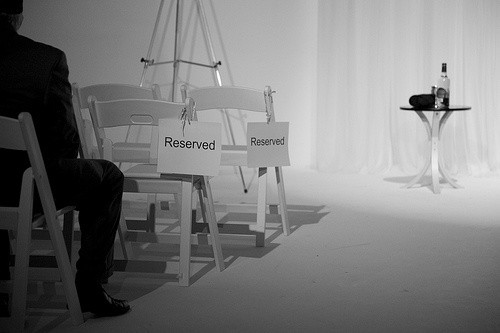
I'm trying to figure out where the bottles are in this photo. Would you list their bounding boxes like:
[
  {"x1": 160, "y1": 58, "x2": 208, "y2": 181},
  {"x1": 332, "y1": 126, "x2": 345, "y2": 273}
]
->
[{"x1": 437, "y1": 62, "x2": 450, "y2": 110}]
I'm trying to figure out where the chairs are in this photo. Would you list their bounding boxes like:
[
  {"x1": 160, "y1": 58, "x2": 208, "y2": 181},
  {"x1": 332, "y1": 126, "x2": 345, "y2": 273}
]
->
[{"x1": 0, "y1": 83, "x2": 291, "y2": 333}]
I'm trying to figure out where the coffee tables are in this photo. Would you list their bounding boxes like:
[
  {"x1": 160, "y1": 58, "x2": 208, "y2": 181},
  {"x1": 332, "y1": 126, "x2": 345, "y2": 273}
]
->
[{"x1": 400, "y1": 105, "x2": 472, "y2": 194}]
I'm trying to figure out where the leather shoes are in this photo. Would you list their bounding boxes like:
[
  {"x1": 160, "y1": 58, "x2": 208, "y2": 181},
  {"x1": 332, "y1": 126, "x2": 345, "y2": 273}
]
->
[{"x1": 66, "y1": 282, "x2": 130, "y2": 316}]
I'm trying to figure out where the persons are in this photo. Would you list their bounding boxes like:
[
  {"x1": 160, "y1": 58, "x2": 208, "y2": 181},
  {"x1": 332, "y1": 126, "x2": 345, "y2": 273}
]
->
[{"x1": 0, "y1": 0, "x2": 131, "y2": 318}]
